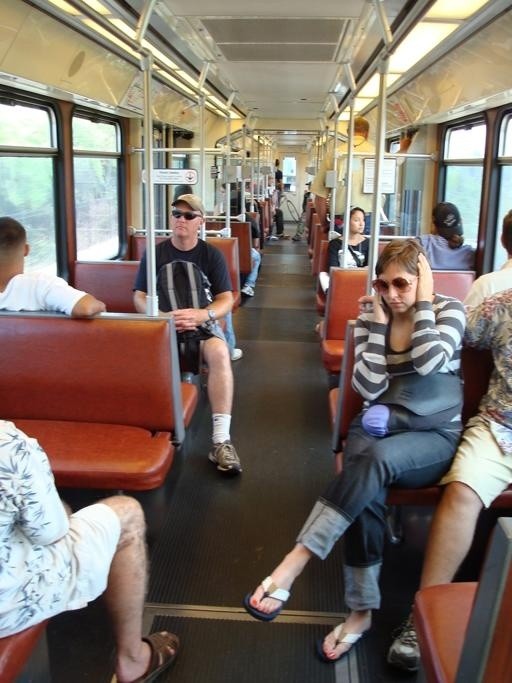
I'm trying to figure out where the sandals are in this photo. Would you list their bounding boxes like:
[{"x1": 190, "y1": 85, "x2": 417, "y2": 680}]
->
[{"x1": 116, "y1": 631, "x2": 181, "y2": 683}]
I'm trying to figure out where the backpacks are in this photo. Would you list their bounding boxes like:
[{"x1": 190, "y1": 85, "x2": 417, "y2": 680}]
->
[{"x1": 158, "y1": 260, "x2": 215, "y2": 376}]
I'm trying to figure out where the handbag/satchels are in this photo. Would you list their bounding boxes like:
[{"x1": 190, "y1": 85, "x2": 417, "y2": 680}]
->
[{"x1": 361, "y1": 372, "x2": 465, "y2": 438}]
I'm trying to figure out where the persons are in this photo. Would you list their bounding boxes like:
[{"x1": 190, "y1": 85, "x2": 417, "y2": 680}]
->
[
  {"x1": 415, "y1": 202, "x2": 476, "y2": 269},
  {"x1": 2, "y1": 217, "x2": 106, "y2": 317},
  {"x1": 132, "y1": 193, "x2": 242, "y2": 472},
  {"x1": 291, "y1": 181, "x2": 311, "y2": 241},
  {"x1": 211, "y1": 168, "x2": 284, "y2": 296},
  {"x1": 358, "y1": 289, "x2": 512, "y2": 673},
  {"x1": 1, "y1": 419, "x2": 179, "y2": 682},
  {"x1": 244, "y1": 236, "x2": 466, "y2": 663},
  {"x1": 462, "y1": 209, "x2": 512, "y2": 305},
  {"x1": 327, "y1": 206, "x2": 369, "y2": 276}
]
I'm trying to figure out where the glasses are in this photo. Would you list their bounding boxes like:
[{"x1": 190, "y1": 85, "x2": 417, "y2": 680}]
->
[
  {"x1": 172, "y1": 210, "x2": 201, "y2": 220},
  {"x1": 370, "y1": 277, "x2": 419, "y2": 293}
]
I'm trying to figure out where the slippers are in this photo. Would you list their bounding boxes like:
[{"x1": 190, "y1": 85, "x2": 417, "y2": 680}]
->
[
  {"x1": 242, "y1": 576, "x2": 293, "y2": 621},
  {"x1": 315, "y1": 622, "x2": 364, "y2": 664}
]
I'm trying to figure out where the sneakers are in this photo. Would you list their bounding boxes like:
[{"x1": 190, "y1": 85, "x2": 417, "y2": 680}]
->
[
  {"x1": 231, "y1": 348, "x2": 243, "y2": 361},
  {"x1": 241, "y1": 283, "x2": 255, "y2": 297},
  {"x1": 292, "y1": 234, "x2": 301, "y2": 241},
  {"x1": 207, "y1": 439, "x2": 243, "y2": 473},
  {"x1": 387, "y1": 612, "x2": 421, "y2": 671}
]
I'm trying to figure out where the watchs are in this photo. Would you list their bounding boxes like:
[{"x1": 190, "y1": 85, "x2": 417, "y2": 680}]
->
[{"x1": 207, "y1": 307, "x2": 216, "y2": 320}]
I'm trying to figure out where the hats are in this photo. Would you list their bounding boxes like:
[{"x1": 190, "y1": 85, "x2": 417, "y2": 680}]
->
[
  {"x1": 171, "y1": 194, "x2": 205, "y2": 215},
  {"x1": 305, "y1": 182, "x2": 311, "y2": 185},
  {"x1": 432, "y1": 202, "x2": 462, "y2": 236}
]
